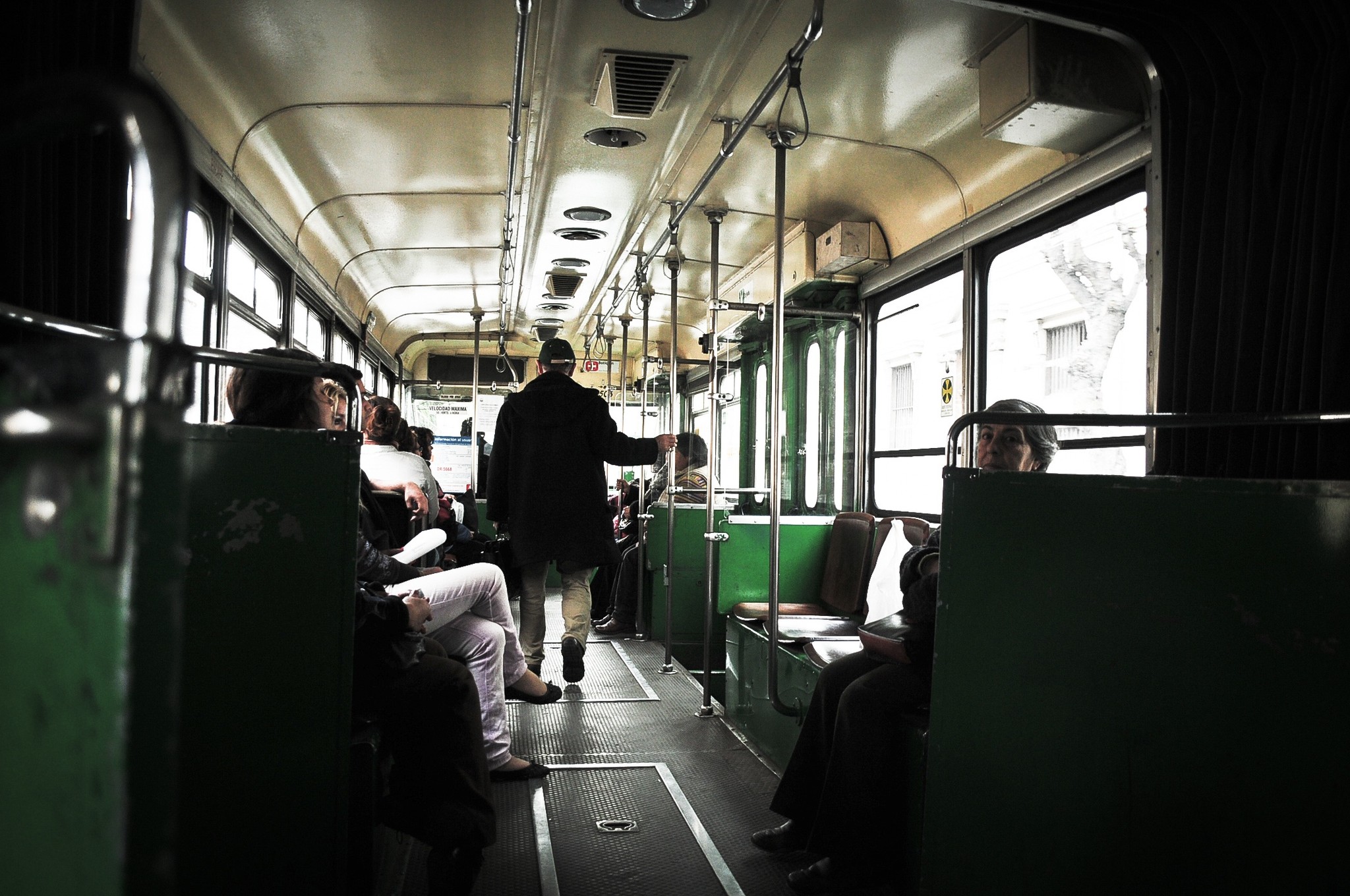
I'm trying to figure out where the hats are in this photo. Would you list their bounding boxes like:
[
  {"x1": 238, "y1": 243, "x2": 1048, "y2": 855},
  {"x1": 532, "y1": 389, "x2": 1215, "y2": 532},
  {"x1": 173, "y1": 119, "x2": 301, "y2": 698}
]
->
[{"x1": 539, "y1": 339, "x2": 576, "y2": 365}]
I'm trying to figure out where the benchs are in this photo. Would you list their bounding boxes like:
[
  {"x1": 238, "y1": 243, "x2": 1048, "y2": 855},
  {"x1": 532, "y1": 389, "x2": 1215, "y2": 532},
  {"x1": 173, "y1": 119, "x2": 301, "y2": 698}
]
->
[
  {"x1": 643, "y1": 410, "x2": 1349, "y2": 896},
  {"x1": 180, "y1": 347, "x2": 425, "y2": 896}
]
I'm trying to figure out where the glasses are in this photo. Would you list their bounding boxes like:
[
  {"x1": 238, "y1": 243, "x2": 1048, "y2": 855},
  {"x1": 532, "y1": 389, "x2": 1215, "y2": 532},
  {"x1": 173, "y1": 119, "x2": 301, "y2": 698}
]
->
[{"x1": 416, "y1": 446, "x2": 423, "y2": 452}]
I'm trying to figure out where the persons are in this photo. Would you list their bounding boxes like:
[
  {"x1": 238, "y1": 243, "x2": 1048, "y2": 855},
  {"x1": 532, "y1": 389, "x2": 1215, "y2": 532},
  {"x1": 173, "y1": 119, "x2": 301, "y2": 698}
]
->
[
  {"x1": 589, "y1": 433, "x2": 724, "y2": 634},
  {"x1": 407, "y1": 434, "x2": 476, "y2": 566},
  {"x1": 393, "y1": 418, "x2": 414, "y2": 451},
  {"x1": 750, "y1": 399, "x2": 1064, "y2": 896},
  {"x1": 418, "y1": 427, "x2": 434, "y2": 460},
  {"x1": 225, "y1": 348, "x2": 496, "y2": 896},
  {"x1": 363, "y1": 396, "x2": 446, "y2": 567},
  {"x1": 460, "y1": 417, "x2": 493, "y2": 499},
  {"x1": 485, "y1": 338, "x2": 677, "y2": 681},
  {"x1": 320, "y1": 379, "x2": 562, "y2": 780}
]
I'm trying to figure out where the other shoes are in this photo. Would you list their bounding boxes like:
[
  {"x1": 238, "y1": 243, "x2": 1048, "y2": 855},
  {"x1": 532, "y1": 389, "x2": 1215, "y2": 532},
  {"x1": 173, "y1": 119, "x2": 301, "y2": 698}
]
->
[
  {"x1": 491, "y1": 761, "x2": 550, "y2": 782},
  {"x1": 527, "y1": 664, "x2": 540, "y2": 675},
  {"x1": 562, "y1": 637, "x2": 584, "y2": 683},
  {"x1": 505, "y1": 680, "x2": 562, "y2": 704},
  {"x1": 426, "y1": 848, "x2": 484, "y2": 896},
  {"x1": 591, "y1": 614, "x2": 605, "y2": 625},
  {"x1": 590, "y1": 608, "x2": 602, "y2": 619}
]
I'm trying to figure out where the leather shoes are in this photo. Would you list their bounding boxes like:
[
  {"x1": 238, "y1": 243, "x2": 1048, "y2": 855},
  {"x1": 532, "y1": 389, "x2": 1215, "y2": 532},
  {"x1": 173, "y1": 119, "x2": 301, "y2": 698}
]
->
[
  {"x1": 786, "y1": 857, "x2": 832, "y2": 893},
  {"x1": 751, "y1": 819, "x2": 820, "y2": 853},
  {"x1": 594, "y1": 618, "x2": 636, "y2": 635},
  {"x1": 593, "y1": 614, "x2": 612, "y2": 627}
]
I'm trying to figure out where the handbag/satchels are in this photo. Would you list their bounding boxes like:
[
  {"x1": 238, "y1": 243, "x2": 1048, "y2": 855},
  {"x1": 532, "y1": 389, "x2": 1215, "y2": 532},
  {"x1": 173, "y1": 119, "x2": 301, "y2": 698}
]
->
[
  {"x1": 481, "y1": 527, "x2": 523, "y2": 599},
  {"x1": 618, "y1": 518, "x2": 634, "y2": 533},
  {"x1": 858, "y1": 609, "x2": 935, "y2": 665}
]
